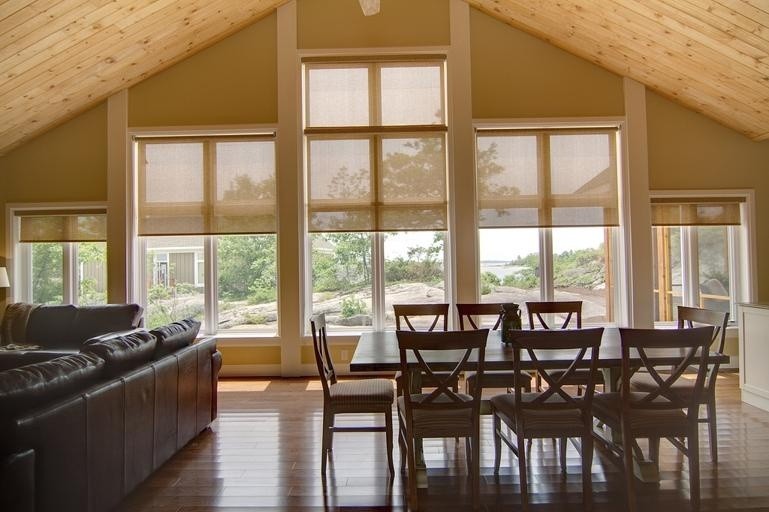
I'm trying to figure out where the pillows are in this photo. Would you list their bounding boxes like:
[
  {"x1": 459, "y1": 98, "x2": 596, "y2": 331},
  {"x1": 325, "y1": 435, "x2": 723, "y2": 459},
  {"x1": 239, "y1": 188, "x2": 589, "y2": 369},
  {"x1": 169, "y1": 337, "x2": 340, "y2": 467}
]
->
[{"x1": 76, "y1": 326, "x2": 111, "y2": 345}]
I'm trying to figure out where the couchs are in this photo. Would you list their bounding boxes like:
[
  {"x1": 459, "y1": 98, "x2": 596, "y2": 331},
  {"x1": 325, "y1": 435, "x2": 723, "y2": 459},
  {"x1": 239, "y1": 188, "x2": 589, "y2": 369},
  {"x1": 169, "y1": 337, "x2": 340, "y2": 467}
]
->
[
  {"x1": 0, "y1": 319, "x2": 223, "y2": 511},
  {"x1": 0, "y1": 302, "x2": 144, "y2": 370}
]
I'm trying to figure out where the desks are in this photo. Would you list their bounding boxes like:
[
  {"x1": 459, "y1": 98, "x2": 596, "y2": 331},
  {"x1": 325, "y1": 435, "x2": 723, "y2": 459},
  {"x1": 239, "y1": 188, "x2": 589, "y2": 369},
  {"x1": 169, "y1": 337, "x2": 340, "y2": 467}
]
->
[{"x1": 351, "y1": 326, "x2": 727, "y2": 490}]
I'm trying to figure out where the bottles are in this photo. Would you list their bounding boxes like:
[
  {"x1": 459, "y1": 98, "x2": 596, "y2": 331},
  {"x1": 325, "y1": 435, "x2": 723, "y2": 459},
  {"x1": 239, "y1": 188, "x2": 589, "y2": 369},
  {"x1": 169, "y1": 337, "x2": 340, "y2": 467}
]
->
[{"x1": 498, "y1": 304, "x2": 521, "y2": 342}]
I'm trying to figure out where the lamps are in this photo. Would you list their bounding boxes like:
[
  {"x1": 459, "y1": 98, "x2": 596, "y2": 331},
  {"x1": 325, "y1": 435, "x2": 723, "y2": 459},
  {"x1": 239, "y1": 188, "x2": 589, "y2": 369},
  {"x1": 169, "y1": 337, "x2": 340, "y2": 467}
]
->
[{"x1": 0, "y1": 267, "x2": 10, "y2": 288}]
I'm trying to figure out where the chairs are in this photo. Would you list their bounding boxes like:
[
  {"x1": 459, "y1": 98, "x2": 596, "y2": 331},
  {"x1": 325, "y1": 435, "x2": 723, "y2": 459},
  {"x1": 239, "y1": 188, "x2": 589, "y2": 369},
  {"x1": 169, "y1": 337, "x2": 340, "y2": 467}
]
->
[
  {"x1": 619, "y1": 305, "x2": 728, "y2": 476},
  {"x1": 456, "y1": 302, "x2": 534, "y2": 459},
  {"x1": 393, "y1": 327, "x2": 489, "y2": 511},
  {"x1": 525, "y1": 302, "x2": 606, "y2": 442},
  {"x1": 488, "y1": 326, "x2": 607, "y2": 510},
  {"x1": 581, "y1": 325, "x2": 716, "y2": 510},
  {"x1": 309, "y1": 311, "x2": 396, "y2": 481},
  {"x1": 391, "y1": 303, "x2": 461, "y2": 443}
]
